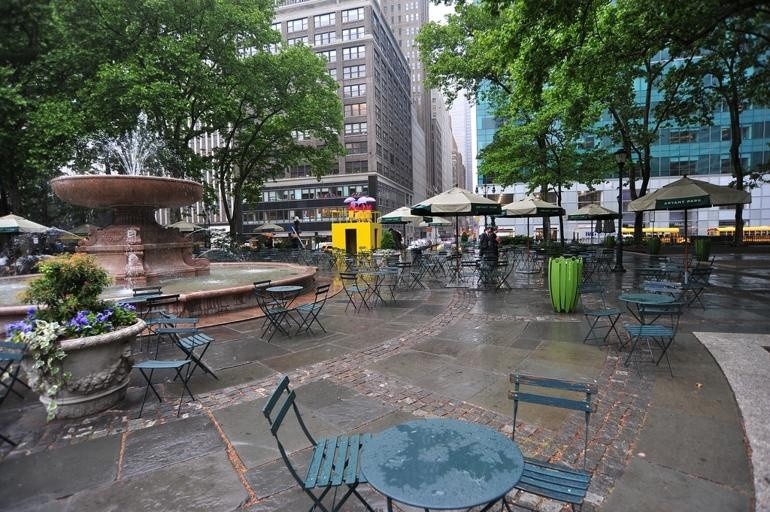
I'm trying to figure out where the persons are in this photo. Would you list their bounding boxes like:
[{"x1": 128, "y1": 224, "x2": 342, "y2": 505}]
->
[
  {"x1": 478, "y1": 225, "x2": 500, "y2": 258},
  {"x1": 388, "y1": 228, "x2": 403, "y2": 250}
]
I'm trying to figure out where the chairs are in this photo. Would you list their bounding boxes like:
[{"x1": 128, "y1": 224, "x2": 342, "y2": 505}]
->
[
  {"x1": 254, "y1": 280, "x2": 291, "y2": 329},
  {"x1": 677, "y1": 267, "x2": 711, "y2": 310},
  {"x1": 0, "y1": 341, "x2": 27, "y2": 404},
  {"x1": 160, "y1": 312, "x2": 219, "y2": 383},
  {"x1": 339, "y1": 271, "x2": 371, "y2": 314},
  {"x1": 133, "y1": 286, "x2": 163, "y2": 345},
  {"x1": 132, "y1": 317, "x2": 198, "y2": 416},
  {"x1": 295, "y1": 283, "x2": 332, "y2": 337},
  {"x1": 618, "y1": 292, "x2": 676, "y2": 346},
  {"x1": 252, "y1": 288, "x2": 294, "y2": 342},
  {"x1": 619, "y1": 304, "x2": 683, "y2": 378},
  {"x1": 261, "y1": 375, "x2": 373, "y2": 510},
  {"x1": 375, "y1": 268, "x2": 398, "y2": 309},
  {"x1": 505, "y1": 372, "x2": 598, "y2": 511},
  {"x1": 578, "y1": 286, "x2": 624, "y2": 349},
  {"x1": 138, "y1": 294, "x2": 181, "y2": 350}
]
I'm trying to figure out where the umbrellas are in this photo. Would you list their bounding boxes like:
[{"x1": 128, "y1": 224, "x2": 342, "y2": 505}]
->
[
  {"x1": 377, "y1": 207, "x2": 432, "y2": 246},
  {"x1": 411, "y1": 185, "x2": 501, "y2": 269},
  {"x1": 0, "y1": 212, "x2": 50, "y2": 274},
  {"x1": 567, "y1": 202, "x2": 625, "y2": 259},
  {"x1": 490, "y1": 193, "x2": 566, "y2": 268},
  {"x1": 253, "y1": 222, "x2": 284, "y2": 233},
  {"x1": 167, "y1": 220, "x2": 204, "y2": 233},
  {"x1": 627, "y1": 174, "x2": 752, "y2": 287},
  {"x1": 31, "y1": 225, "x2": 84, "y2": 244},
  {"x1": 418, "y1": 216, "x2": 453, "y2": 245}
]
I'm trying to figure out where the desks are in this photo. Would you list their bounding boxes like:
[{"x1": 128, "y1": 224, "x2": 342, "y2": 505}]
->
[
  {"x1": 265, "y1": 285, "x2": 301, "y2": 330},
  {"x1": 122, "y1": 295, "x2": 180, "y2": 352},
  {"x1": 359, "y1": 418, "x2": 524, "y2": 511},
  {"x1": 360, "y1": 272, "x2": 387, "y2": 306}
]
